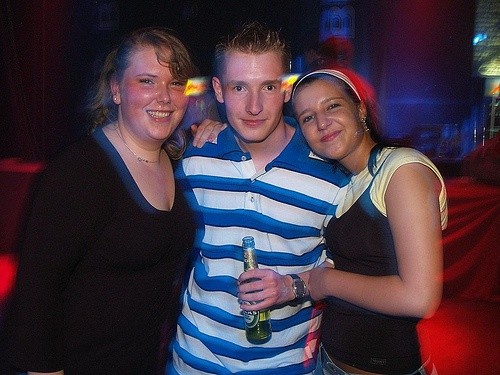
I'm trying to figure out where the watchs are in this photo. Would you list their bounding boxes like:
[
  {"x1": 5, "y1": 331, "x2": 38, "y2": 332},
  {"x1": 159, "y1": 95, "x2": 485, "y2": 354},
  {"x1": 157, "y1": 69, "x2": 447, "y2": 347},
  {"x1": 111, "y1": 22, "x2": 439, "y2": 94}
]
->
[{"x1": 285, "y1": 273, "x2": 307, "y2": 307}]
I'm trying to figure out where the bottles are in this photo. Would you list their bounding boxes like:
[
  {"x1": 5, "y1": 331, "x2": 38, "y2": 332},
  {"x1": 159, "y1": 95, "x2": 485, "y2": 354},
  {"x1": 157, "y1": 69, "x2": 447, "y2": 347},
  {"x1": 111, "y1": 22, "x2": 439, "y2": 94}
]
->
[{"x1": 243, "y1": 235, "x2": 272, "y2": 344}]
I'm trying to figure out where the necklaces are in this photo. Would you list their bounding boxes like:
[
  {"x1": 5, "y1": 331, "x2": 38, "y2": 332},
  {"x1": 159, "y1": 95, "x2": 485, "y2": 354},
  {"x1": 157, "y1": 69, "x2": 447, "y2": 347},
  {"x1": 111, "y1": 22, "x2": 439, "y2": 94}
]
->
[{"x1": 112, "y1": 118, "x2": 160, "y2": 164}]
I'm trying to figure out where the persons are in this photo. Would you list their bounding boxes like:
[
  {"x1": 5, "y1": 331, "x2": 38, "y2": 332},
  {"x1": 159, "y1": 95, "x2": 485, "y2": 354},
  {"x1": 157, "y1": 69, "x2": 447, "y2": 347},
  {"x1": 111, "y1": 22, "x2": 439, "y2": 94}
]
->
[
  {"x1": 0, "y1": 26, "x2": 229, "y2": 375},
  {"x1": 290, "y1": 62, "x2": 450, "y2": 375},
  {"x1": 176, "y1": 22, "x2": 354, "y2": 375}
]
